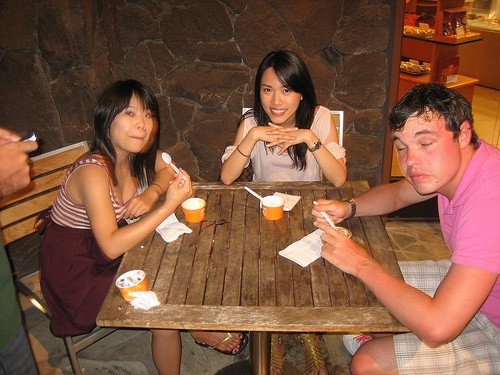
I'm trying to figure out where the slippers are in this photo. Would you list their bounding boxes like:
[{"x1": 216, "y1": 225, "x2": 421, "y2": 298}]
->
[{"x1": 195, "y1": 330, "x2": 248, "y2": 355}]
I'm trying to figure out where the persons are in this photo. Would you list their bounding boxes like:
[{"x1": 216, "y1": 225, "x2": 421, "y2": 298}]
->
[
  {"x1": 220, "y1": 49, "x2": 348, "y2": 189},
  {"x1": 38, "y1": 79, "x2": 249, "y2": 375},
  {"x1": 0, "y1": 127, "x2": 39, "y2": 375},
  {"x1": 310, "y1": 81, "x2": 500, "y2": 375}
]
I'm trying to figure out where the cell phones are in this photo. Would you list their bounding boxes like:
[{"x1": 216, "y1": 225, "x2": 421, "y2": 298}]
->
[{"x1": 20, "y1": 133, "x2": 37, "y2": 142}]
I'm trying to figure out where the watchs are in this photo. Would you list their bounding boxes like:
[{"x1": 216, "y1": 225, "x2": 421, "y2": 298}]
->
[{"x1": 309, "y1": 140, "x2": 322, "y2": 153}]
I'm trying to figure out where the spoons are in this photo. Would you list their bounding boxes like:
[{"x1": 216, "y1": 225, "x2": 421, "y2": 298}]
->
[{"x1": 161, "y1": 152, "x2": 186, "y2": 180}]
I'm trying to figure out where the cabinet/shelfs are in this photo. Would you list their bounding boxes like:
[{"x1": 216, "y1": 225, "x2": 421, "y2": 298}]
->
[{"x1": 397, "y1": 0, "x2": 482, "y2": 103}]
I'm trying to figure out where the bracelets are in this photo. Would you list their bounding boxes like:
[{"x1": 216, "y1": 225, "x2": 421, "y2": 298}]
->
[
  {"x1": 150, "y1": 182, "x2": 164, "y2": 193},
  {"x1": 341, "y1": 197, "x2": 357, "y2": 219},
  {"x1": 236, "y1": 145, "x2": 251, "y2": 158}
]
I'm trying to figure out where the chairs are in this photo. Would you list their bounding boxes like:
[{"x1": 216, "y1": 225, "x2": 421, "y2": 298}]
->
[
  {"x1": 0, "y1": 140, "x2": 119, "y2": 375},
  {"x1": 242, "y1": 107, "x2": 344, "y2": 146}
]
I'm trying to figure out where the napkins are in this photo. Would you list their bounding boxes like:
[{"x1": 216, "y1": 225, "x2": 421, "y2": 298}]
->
[
  {"x1": 128, "y1": 291, "x2": 160, "y2": 311},
  {"x1": 272, "y1": 192, "x2": 301, "y2": 211},
  {"x1": 155, "y1": 213, "x2": 193, "y2": 244},
  {"x1": 278, "y1": 229, "x2": 324, "y2": 268}
]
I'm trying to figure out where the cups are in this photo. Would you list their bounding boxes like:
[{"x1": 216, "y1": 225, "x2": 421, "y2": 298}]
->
[
  {"x1": 181, "y1": 197, "x2": 206, "y2": 223},
  {"x1": 261, "y1": 196, "x2": 284, "y2": 220},
  {"x1": 115, "y1": 270, "x2": 147, "y2": 303},
  {"x1": 325, "y1": 227, "x2": 352, "y2": 246}
]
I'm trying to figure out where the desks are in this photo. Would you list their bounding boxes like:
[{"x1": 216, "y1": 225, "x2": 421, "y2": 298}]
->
[{"x1": 96, "y1": 182, "x2": 411, "y2": 375}]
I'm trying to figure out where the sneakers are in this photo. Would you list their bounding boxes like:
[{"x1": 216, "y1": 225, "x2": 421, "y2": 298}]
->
[{"x1": 343, "y1": 333, "x2": 373, "y2": 356}]
